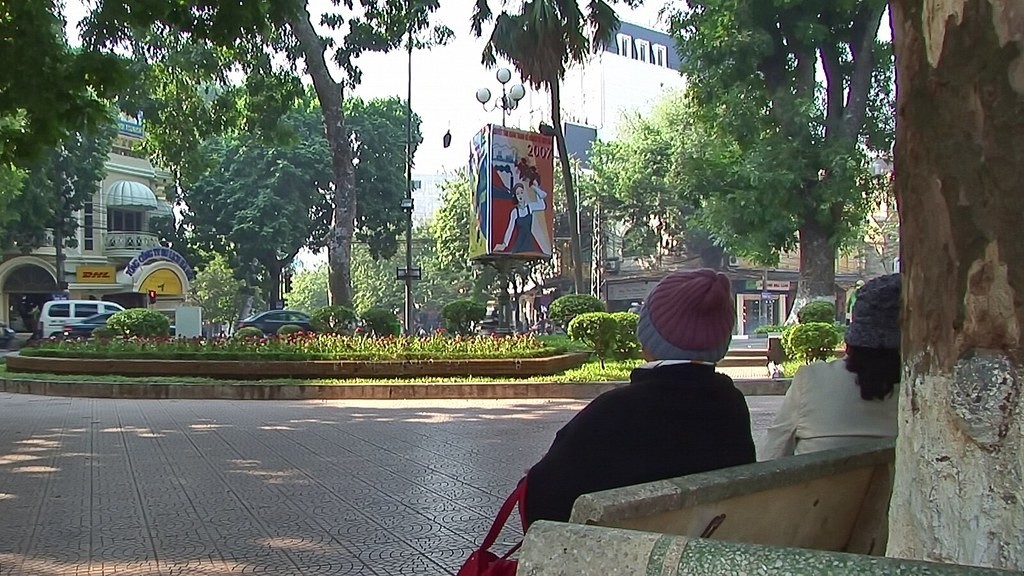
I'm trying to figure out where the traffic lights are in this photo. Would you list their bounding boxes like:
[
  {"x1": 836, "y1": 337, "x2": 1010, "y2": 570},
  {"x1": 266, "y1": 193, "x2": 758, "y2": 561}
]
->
[
  {"x1": 149, "y1": 290, "x2": 157, "y2": 305},
  {"x1": 284, "y1": 273, "x2": 293, "y2": 293}
]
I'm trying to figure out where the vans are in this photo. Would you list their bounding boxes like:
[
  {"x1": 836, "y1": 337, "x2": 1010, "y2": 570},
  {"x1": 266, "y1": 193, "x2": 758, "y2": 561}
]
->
[{"x1": 35, "y1": 298, "x2": 128, "y2": 341}]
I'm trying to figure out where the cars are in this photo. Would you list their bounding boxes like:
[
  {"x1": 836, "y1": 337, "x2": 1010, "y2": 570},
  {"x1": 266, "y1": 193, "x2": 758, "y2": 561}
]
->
[
  {"x1": 0, "y1": 321, "x2": 15, "y2": 345},
  {"x1": 234, "y1": 309, "x2": 313, "y2": 337},
  {"x1": 61, "y1": 313, "x2": 113, "y2": 342},
  {"x1": 470, "y1": 318, "x2": 516, "y2": 338}
]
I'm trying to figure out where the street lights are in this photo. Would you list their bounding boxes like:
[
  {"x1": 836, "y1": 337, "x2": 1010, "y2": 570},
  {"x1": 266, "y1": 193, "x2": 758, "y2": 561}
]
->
[{"x1": 475, "y1": 67, "x2": 526, "y2": 126}]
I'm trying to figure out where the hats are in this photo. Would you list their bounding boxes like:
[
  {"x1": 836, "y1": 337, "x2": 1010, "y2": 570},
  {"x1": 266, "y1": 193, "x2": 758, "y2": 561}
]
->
[
  {"x1": 636, "y1": 268, "x2": 735, "y2": 362},
  {"x1": 845, "y1": 273, "x2": 902, "y2": 348}
]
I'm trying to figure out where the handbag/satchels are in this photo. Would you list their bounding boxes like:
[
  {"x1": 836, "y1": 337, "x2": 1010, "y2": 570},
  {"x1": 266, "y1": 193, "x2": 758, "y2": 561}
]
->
[{"x1": 455, "y1": 475, "x2": 528, "y2": 576}]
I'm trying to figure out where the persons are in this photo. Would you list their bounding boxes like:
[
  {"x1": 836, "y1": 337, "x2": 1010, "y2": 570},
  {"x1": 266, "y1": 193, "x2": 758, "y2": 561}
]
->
[
  {"x1": 518, "y1": 269, "x2": 757, "y2": 536},
  {"x1": 756, "y1": 273, "x2": 902, "y2": 462},
  {"x1": 846, "y1": 280, "x2": 866, "y2": 313}
]
